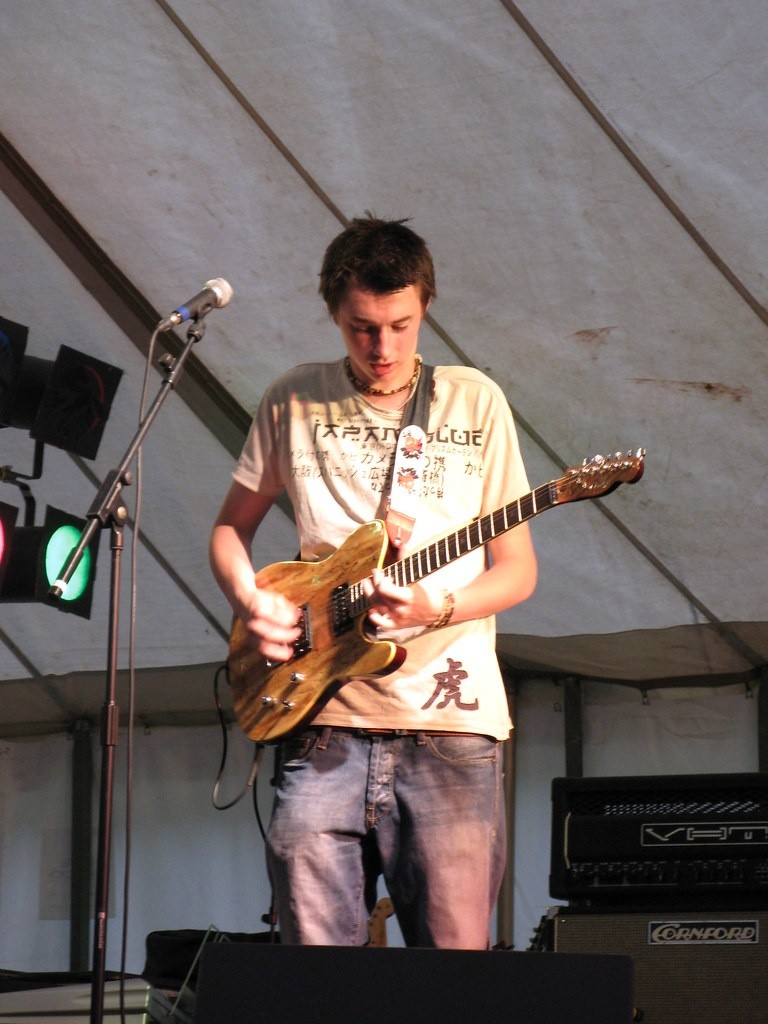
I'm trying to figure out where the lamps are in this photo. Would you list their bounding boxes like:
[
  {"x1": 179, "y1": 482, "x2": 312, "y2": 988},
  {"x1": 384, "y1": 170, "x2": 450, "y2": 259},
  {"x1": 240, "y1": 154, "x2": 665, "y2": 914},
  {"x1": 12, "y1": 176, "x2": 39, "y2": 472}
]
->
[
  {"x1": 0, "y1": 501, "x2": 101, "y2": 619},
  {"x1": 0, "y1": 316, "x2": 125, "y2": 461}
]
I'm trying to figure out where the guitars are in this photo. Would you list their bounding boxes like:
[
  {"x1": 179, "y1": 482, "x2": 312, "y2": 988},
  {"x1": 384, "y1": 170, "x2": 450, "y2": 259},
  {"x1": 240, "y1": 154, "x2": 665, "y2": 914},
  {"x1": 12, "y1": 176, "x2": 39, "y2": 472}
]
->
[{"x1": 226, "y1": 447, "x2": 648, "y2": 745}]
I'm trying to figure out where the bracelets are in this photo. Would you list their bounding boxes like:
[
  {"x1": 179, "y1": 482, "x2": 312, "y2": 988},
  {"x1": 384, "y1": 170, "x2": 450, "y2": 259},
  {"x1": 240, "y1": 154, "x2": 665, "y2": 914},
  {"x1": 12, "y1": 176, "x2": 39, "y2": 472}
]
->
[{"x1": 428, "y1": 588, "x2": 455, "y2": 630}]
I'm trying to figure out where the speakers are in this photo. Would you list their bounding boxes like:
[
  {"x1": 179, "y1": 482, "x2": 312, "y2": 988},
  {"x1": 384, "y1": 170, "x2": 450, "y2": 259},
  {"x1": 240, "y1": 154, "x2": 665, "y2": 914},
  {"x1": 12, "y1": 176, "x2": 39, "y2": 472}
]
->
[
  {"x1": 545, "y1": 903, "x2": 768, "y2": 1024},
  {"x1": 194, "y1": 941, "x2": 635, "y2": 1024}
]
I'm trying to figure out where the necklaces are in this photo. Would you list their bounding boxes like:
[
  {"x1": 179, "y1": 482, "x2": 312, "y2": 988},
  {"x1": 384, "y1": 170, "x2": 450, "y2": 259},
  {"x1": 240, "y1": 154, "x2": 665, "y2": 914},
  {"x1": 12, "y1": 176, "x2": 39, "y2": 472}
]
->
[{"x1": 344, "y1": 354, "x2": 420, "y2": 397}]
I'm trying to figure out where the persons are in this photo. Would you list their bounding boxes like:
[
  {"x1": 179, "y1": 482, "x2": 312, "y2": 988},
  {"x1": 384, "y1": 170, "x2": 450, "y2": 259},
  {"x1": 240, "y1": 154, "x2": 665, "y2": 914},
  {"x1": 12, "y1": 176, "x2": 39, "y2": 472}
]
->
[{"x1": 208, "y1": 209, "x2": 539, "y2": 950}]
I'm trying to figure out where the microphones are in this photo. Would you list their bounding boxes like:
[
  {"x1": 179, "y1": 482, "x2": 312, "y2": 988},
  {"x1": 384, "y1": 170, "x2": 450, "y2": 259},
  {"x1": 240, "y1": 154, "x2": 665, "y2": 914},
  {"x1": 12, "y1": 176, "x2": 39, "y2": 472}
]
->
[{"x1": 158, "y1": 278, "x2": 232, "y2": 332}]
